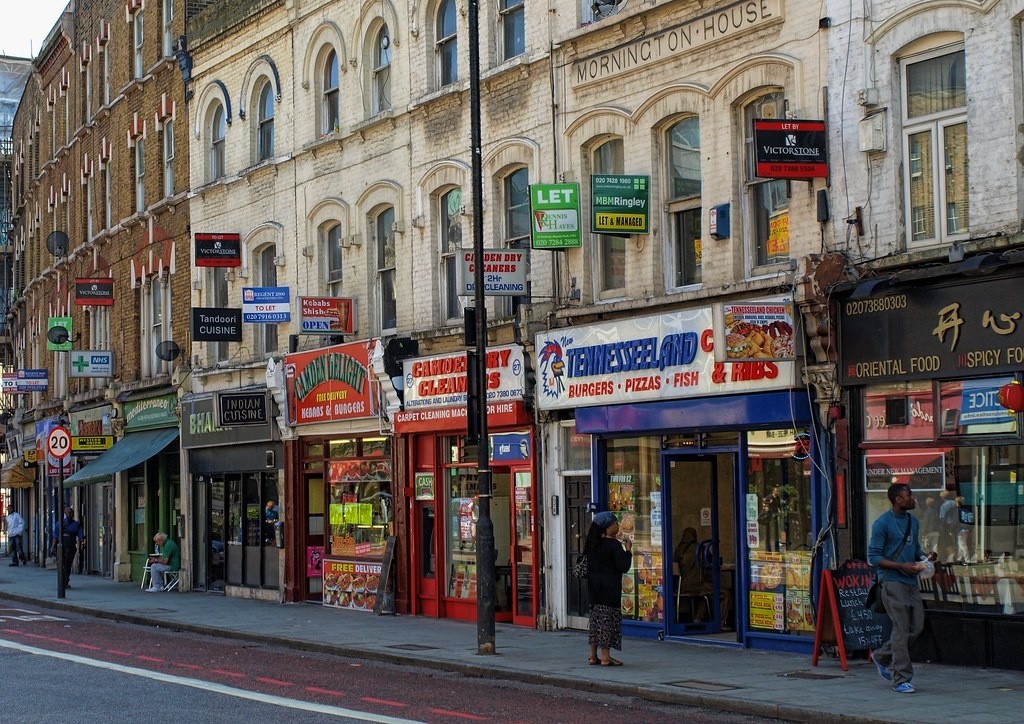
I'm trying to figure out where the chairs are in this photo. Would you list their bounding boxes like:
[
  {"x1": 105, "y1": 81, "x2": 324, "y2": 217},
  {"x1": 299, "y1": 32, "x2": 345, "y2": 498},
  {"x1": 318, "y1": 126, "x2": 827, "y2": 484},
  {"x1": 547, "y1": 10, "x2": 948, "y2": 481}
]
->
[
  {"x1": 141, "y1": 558, "x2": 188, "y2": 592},
  {"x1": 917, "y1": 561, "x2": 1000, "y2": 605},
  {"x1": 673, "y1": 562, "x2": 712, "y2": 623}
]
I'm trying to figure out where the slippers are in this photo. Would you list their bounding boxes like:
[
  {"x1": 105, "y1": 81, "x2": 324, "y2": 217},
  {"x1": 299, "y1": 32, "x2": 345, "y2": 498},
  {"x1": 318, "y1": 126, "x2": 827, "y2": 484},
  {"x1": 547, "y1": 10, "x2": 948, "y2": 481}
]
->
[
  {"x1": 600, "y1": 657, "x2": 623, "y2": 666},
  {"x1": 588, "y1": 656, "x2": 601, "y2": 664}
]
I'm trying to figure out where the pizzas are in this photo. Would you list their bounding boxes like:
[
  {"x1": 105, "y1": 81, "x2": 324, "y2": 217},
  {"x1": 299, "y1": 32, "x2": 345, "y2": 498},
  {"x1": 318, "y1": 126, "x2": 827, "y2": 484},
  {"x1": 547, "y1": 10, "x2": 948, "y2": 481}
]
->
[{"x1": 760, "y1": 564, "x2": 783, "y2": 588}]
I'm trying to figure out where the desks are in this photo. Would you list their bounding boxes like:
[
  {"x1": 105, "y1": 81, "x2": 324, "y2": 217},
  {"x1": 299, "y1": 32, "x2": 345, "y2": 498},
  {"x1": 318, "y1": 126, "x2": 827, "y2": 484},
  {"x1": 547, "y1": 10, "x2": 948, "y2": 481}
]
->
[
  {"x1": 495, "y1": 565, "x2": 512, "y2": 576},
  {"x1": 149, "y1": 553, "x2": 167, "y2": 586}
]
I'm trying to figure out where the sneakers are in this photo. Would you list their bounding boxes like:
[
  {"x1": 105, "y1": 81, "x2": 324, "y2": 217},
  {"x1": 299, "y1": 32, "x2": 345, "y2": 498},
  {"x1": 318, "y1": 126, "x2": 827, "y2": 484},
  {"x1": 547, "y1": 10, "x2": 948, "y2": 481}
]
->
[
  {"x1": 892, "y1": 680, "x2": 916, "y2": 693},
  {"x1": 871, "y1": 652, "x2": 893, "y2": 680}
]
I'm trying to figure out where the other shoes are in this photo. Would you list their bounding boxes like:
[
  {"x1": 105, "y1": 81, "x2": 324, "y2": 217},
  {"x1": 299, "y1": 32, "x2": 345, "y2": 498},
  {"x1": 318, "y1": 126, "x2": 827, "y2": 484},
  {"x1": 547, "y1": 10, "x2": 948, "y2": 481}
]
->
[
  {"x1": 722, "y1": 625, "x2": 732, "y2": 631},
  {"x1": 22, "y1": 559, "x2": 27, "y2": 565},
  {"x1": 146, "y1": 585, "x2": 164, "y2": 592},
  {"x1": 9, "y1": 563, "x2": 20, "y2": 567}
]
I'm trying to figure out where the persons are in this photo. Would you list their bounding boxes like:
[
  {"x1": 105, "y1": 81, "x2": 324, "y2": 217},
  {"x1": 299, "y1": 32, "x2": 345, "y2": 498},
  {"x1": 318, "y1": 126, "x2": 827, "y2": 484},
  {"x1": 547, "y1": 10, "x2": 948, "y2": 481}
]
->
[
  {"x1": 576, "y1": 512, "x2": 632, "y2": 668},
  {"x1": 674, "y1": 527, "x2": 713, "y2": 624},
  {"x1": 868, "y1": 483, "x2": 937, "y2": 693},
  {"x1": 144, "y1": 532, "x2": 181, "y2": 593},
  {"x1": 6, "y1": 505, "x2": 27, "y2": 567},
  {"x1": 912, "y1": 488, "x2": 974, "y2": 564},
  {"x1": 49, "y1": 507, "x2": 84, "y2": 588},
  {"x1": 265, "y1": 501, "x2": 279, "y2": 546}
]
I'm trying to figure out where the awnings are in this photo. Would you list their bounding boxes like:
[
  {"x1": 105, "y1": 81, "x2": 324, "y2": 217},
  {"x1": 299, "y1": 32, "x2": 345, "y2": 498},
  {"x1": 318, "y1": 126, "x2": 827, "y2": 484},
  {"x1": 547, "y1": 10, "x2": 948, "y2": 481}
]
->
[
  {"x1": 1, "y1": 455, "x2": 36, "y2": 488},
  {"x1": 803, "y1": 447, "x2": 953, "y2": 481},
  {"x1": 63, "y1": 427, "x2": 180, "y2": 490}
]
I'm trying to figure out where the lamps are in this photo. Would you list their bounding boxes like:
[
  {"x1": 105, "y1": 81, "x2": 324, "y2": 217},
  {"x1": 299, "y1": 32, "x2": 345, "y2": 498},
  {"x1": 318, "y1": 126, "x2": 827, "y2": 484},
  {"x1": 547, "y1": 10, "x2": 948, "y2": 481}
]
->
[
  {"x1": 949, "y1": 231, "x2": 1002, "y2": 262},
  {"x1": 850, "y1": 274, "x2": 898, "y2": 300},
  {"x1": 265, "y1": 450, "x2": 275, "y2": 468}
]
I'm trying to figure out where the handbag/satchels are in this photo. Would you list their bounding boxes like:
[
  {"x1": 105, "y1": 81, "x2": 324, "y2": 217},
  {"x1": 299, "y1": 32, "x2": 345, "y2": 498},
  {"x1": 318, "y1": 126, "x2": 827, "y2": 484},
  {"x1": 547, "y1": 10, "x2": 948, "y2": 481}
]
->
[
  {"x1": 865, "y1": 582, "x2": 887, "y2": 615},
  {"x1": 572, "y1": 544, "x2": 588, "y2": 577}
]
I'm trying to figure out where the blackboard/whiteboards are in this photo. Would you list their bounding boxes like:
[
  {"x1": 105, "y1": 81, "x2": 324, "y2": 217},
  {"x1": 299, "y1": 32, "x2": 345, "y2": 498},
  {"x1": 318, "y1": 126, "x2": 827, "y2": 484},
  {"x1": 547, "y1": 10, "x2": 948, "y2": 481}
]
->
[
  {"x1": 373, "y1": 535, "x2": 396, "y2": 615},
  {"x1": 816, "y1": 559, "x2": 894, "y2": 648}
]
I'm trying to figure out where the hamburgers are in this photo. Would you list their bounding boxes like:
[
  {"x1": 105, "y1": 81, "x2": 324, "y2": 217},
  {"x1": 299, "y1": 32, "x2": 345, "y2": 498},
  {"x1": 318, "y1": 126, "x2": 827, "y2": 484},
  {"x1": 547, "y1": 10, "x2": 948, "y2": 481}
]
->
[
  {"x1": 640, "y1": 596, "x2": 650, "y2": 610},
  {"x1": 726, "y1": 333, "x2": 750, "y2": 358},
  {"x1": 622, "y1": 576, "x2": 633, "y2": 594},
  {"x1": 621, "y1": 597, "x2": 633, "y2": 613},
  {"x1": 788, "y1": 609, "x2": 803, "y2": 629}
]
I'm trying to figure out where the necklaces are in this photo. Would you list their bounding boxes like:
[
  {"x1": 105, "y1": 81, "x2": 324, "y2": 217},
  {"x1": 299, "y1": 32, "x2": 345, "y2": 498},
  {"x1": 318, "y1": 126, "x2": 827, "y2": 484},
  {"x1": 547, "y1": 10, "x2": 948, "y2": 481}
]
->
[{"x1": 604, "y1": 533, "x2": 627, "y2": 543}]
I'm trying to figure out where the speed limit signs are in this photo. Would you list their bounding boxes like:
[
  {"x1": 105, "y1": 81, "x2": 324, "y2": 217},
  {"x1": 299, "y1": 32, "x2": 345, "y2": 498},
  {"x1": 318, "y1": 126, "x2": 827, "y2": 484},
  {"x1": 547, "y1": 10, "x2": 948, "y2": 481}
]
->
[{"x1": 46, "y1": 426, "x2": 74, "y2": 461}]
